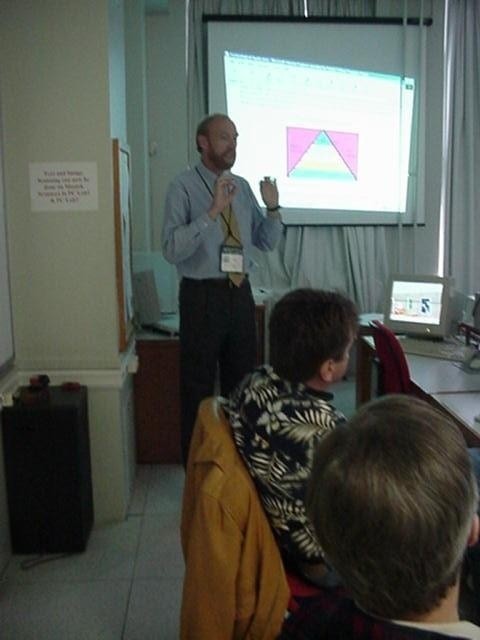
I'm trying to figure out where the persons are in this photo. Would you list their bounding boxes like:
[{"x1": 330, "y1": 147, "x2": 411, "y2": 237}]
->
[
  {"x1": 224, "y1": 289, "x2": 480, "y2": 588},
  {"x1": 275, "y1": 395, "x2": 480, "y2": 640},
  {"x1": 160, "y1": 113, "x2": 284, "y2": 475}
]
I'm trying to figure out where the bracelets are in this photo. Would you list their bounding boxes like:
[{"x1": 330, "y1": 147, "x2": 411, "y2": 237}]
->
[{"x1": 267, "y1": 205, "x2": 282, "y2": 211}]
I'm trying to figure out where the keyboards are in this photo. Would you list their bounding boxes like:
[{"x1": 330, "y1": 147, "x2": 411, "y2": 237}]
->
[{"x1": 397, "y1": 335, "x2": 478, "y2": 364}]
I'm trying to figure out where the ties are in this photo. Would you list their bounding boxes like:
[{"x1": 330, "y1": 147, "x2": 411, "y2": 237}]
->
[{"x1": 221, "y1": 206, "x2": 243, "y2": 289}]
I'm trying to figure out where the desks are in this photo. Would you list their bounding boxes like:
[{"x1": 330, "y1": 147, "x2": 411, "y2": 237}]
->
[{"x1": 354, "y1": 333, "x2": 480, "y2": 448}]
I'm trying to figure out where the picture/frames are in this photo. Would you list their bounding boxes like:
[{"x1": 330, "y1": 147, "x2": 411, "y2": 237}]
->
[{"x1": 112, "y1": 137, "x2": 139, "y2": 353}]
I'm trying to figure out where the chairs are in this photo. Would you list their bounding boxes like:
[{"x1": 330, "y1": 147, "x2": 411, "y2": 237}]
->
[
  {"x1": 368, "y1": 320, "x2": 410, "y2": 396},
  {"x1": 179, "y1": 393, "x2": 319, "y2": 640}
]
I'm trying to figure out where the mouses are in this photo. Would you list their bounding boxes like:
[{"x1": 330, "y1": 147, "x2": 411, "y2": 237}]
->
[{"x1": 469, "y1": 356, "x2": 480, "y2": 371}]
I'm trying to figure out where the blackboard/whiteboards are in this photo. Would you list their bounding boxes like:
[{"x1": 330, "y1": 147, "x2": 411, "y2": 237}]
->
[{"x1": 0, "y1": 117, "x2": 16, "y2": 377}]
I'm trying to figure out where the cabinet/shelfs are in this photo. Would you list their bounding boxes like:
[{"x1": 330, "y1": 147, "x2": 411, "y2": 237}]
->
[{"x1": 136, "y1": 328, "x2": 187, "y2": 465}]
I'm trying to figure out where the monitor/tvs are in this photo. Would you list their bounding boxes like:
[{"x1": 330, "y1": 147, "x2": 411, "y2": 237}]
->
[{"x1": 383, "y1": 274, "x2": 457, "y2": 341}]
